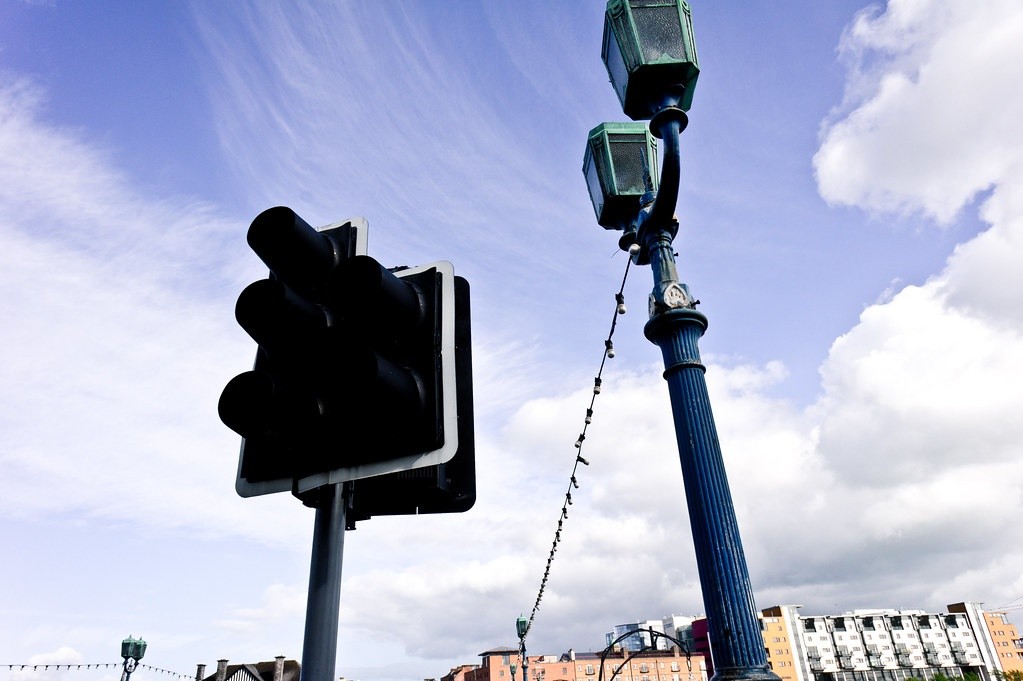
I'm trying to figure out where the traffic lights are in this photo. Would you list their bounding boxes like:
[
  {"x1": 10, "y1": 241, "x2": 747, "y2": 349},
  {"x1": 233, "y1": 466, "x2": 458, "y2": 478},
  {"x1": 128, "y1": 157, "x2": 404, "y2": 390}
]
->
[{"x1": 217, "y1": 205, "x2": 459, "y2": 499}]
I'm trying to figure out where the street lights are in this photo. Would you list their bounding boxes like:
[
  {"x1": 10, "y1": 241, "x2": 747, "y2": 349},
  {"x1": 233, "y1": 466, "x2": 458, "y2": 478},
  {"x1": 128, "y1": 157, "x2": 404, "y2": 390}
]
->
[
  {"x1": 582, "y1": 0, "x2": 783, "y2": 681},
  {"x1": 517, "y1": 614, "x2": 528, "y2": 681},
  {"x1": 510, "y1": 663, "x2": 517, "y2": 681},
  {"x1": 120, "y1": 638, "x2": 146, "y2": 681}
]
